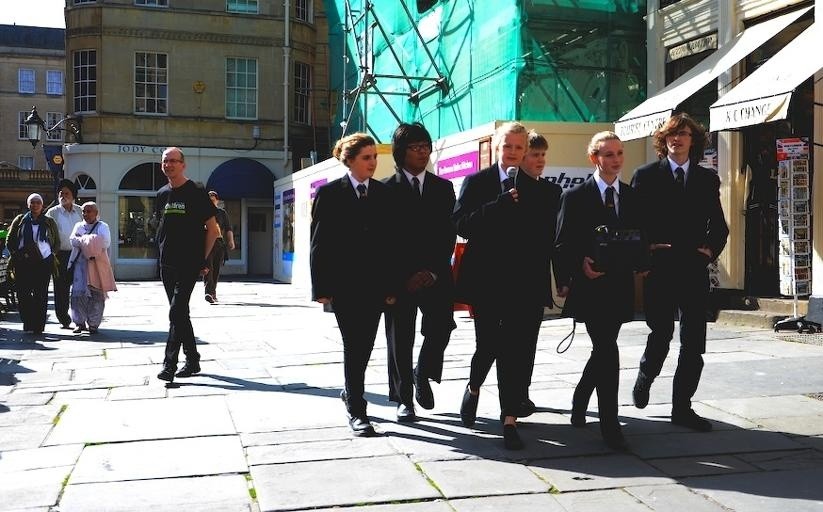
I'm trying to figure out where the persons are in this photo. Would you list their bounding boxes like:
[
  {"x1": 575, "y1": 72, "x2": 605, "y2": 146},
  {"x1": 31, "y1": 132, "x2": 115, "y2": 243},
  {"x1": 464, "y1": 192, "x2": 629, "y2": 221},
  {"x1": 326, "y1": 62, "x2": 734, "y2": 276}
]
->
[
  {"x1": 66, "y1": 200, "x2": 130, "y2": 322},
  {"x1": 450, "y1": 120, "x2": 552, "y2": 450},
  {"x1": 0, "y1": 229, "x2": 10, "y2": 259},
  {"x1": 551, "y1": 130, "x2": 647, "y2": 451},
  {"x1": 203, "y1": 191, "x2": 235, "y2": 303},
  {"x1": 149, "y1": 145, "x2": 221, "y2": 384},
  {"x1": 309, "y1": 132, "x2": 393, "y2": 435},
  {"x1": 517, "y1": 129, "x2": 563, "y2": 414},
  {"x1": 44, "y1": 178, "x2": 83, "y2": 329},
  {"x1": 378, "y1": 123, "x2": 456, "y2": 424},
  {"x1": 630, "y1": 112, "x2": 730, "y2": 431},
  {"x1": 4, "y1": 193, "x2": 61, "y2": 340}
]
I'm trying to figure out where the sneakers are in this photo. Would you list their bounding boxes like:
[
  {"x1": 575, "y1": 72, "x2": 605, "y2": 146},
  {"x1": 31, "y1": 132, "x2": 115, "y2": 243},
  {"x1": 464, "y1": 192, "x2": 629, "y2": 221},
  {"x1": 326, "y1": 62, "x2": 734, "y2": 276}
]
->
[
  {"x1": 211, "y1": 294, "x2": 218, "y2": 302},
  {"x1": 204, "y1": 294, "x2": 214, "y2": 303}
]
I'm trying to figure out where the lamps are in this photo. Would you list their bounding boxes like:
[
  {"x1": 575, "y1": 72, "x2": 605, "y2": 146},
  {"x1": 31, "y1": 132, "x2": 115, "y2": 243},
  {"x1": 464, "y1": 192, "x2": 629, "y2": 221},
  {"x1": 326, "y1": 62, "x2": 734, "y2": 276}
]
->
[
  {"x1": 22, "y1": 104, "x2": 83, "y2": 150},
  {"x1": 252, "y1": 125, "x2": 284, "y2": 143}
]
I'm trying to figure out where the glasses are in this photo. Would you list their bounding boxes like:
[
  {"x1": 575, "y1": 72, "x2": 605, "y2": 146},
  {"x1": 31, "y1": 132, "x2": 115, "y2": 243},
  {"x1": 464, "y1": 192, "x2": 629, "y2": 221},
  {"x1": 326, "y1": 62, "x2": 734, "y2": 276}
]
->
[
  {"x1": 669, "y1": 131, "x2": 691, "y2": 136},
  {"x1": 161, "y1": 159, "x2": 181, "y2": 163},
  {"x1": 407, "y1": 143, "x2": 430, "y2": 152}
]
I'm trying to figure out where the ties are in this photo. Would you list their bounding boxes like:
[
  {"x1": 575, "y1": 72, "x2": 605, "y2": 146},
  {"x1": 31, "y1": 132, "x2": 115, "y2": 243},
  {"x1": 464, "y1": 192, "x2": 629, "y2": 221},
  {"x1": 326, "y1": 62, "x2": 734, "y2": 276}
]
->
[
  {"x1": 674, "y1": 167, "x2": 685, "y2": 192},
  {"x1": 357, "y1": 184, "x2": 365, "y2": 199},
  {"x1": 411, "y1": 176, "x2": 421, "y2": 203},
  {"x1": 503, "y1": 179, "x2": 514, "y2": 192},
  {"x1": 604, "y1": 187, "x2": 617, "y2": 218}
]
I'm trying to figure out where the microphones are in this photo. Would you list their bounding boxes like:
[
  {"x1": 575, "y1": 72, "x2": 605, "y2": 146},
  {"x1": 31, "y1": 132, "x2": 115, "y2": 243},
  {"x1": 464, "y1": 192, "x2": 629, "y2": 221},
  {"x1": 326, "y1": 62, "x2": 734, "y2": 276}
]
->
[{"x1": 507, "y1": 166, "x2": 516, "y2": 190}]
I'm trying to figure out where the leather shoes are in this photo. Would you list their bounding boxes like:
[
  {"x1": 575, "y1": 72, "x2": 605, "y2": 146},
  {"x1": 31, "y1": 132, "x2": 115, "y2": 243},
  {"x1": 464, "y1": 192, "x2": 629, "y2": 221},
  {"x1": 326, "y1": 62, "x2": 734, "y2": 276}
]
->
[
  {"x1": 602, "y1": 430, "x2": 624, "y2": 447},
  {"x1": 412, "y1": 369, "x2": 433, "y2": 409},
  {"x1": 23, "y1": 317, "x2": 97, "y2": 335},
  {"x1": 632, "y1": 377, "x2": 651, "y2": 408},
  {"x1": 570, "y1": 414, "x2": 585, "y2": 427},
  {"x1": 503, "y1": 424, "x2": 519, "y2": 451},
  {"x1": 348, "y1": 415, "x2": 375, "y2": 436},
  {"x1": 459, "y1": 382, "x2": 479, "y2": 428},
  {"x1": 175, "y1": 363, "x2": 200, "y2": 377},
  {"x1": 157, "y1": 367, "x2": 174, "y2": 381},
  {"x1": 670, "y1": 408, "x2": 711, "y2": 431},
  {"x1": 397, "y1": 402, "x2": 414, "y2": 420}
]
